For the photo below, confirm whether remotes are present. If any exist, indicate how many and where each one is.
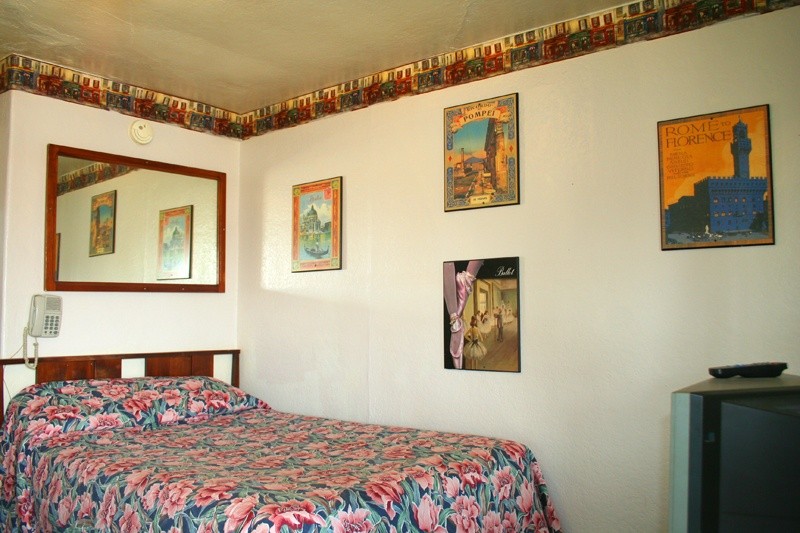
[709,362,788,379]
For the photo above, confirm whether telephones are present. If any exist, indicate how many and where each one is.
[28,294,62,338]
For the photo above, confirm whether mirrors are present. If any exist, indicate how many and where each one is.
[45,143,226,293]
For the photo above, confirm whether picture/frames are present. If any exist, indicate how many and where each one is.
[443,256,521,373]
[442,92,521,212]
[291,176,343,273]
[656,104,776,252]
[89,189,117,257]
[156,204,194,280]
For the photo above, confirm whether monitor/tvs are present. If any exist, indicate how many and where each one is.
[670,371,800,533]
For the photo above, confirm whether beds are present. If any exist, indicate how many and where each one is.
[0,348,567,533]
